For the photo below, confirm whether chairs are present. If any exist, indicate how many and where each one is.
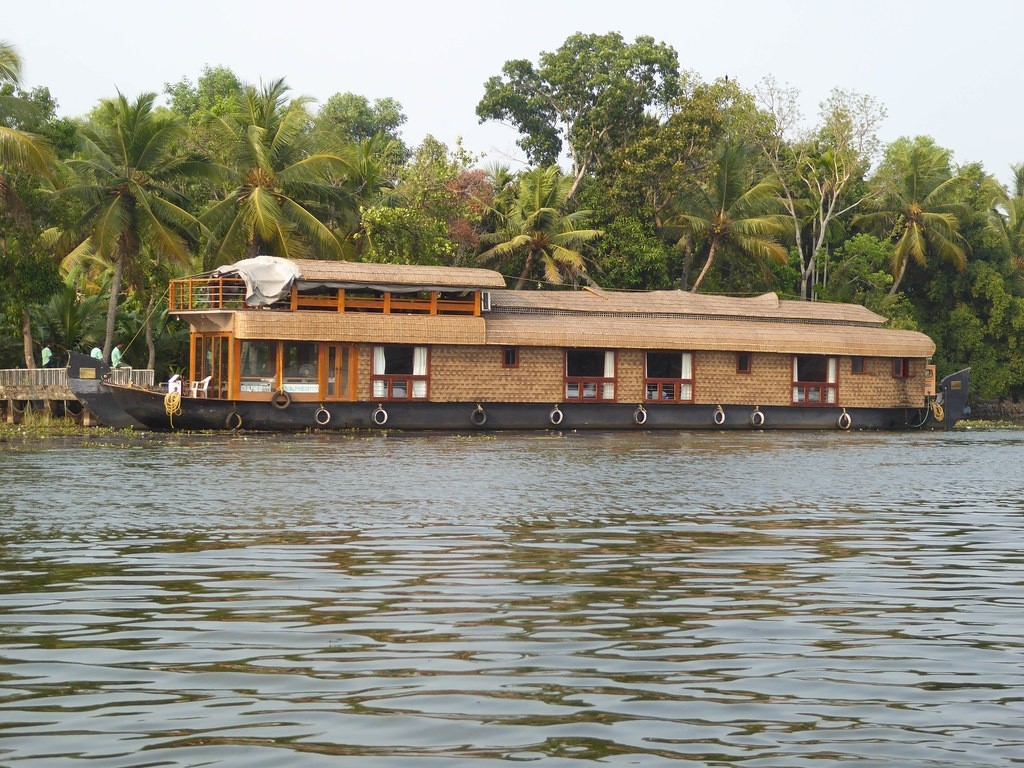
[188,376,213,398]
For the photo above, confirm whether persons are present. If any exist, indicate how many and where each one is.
[42,343,61,369]
[111,342,130,369]
[91,344,104,361]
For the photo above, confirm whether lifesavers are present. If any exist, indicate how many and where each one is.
[224,413,243,430]
[711,410,726,424]
[549,409,564,426]
[471,408,488,427]
[371,410,388,425]
[271,390,292,409]
[836,413,852,430]
[633,407,648,425]
[750,411,765,426]
[313,408,332,426]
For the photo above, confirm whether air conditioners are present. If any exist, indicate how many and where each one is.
[481,292,491,312]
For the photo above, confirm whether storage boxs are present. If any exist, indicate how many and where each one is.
[168,374,183,396]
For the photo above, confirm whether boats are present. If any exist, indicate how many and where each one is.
[53,255,972,431]
